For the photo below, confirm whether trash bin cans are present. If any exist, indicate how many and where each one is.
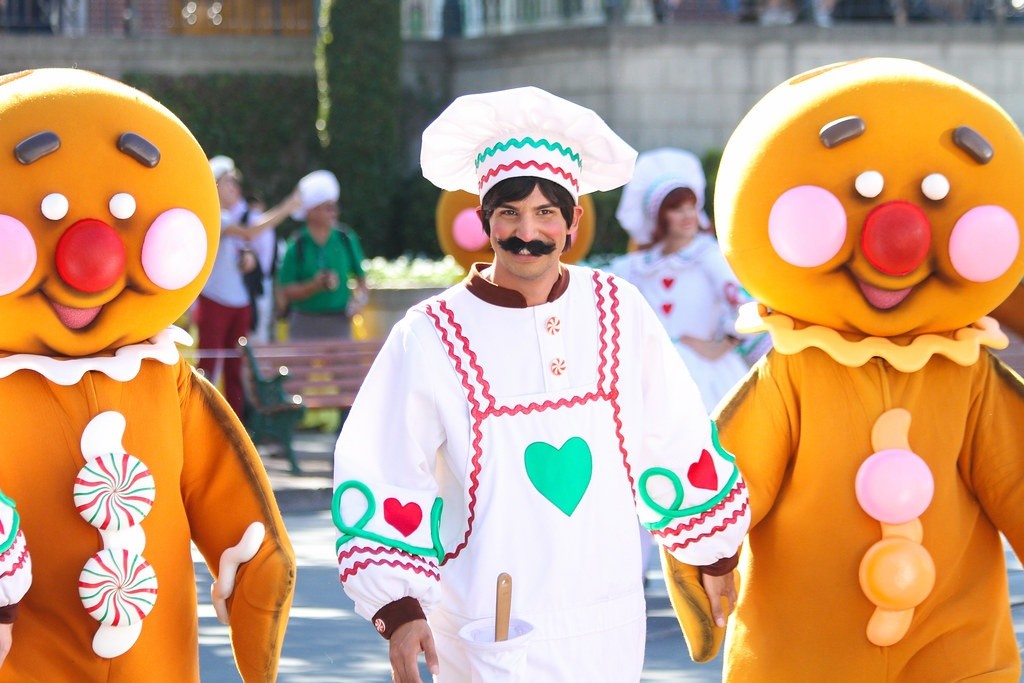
[367,287,449,347]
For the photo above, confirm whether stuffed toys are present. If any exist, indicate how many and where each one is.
[434,186,595,286]
[0,64,298,683]
[660,58,1024,683]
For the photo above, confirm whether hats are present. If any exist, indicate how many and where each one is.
[421,87,640,206]
[615,149,709,245]
[209,156,235,183]
[290,169,340,221]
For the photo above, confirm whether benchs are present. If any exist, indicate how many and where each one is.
[236,335,388,476]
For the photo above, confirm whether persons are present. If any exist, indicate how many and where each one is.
[189,145,369,430]
[604,147,774,422]
[334,87,751,683]
